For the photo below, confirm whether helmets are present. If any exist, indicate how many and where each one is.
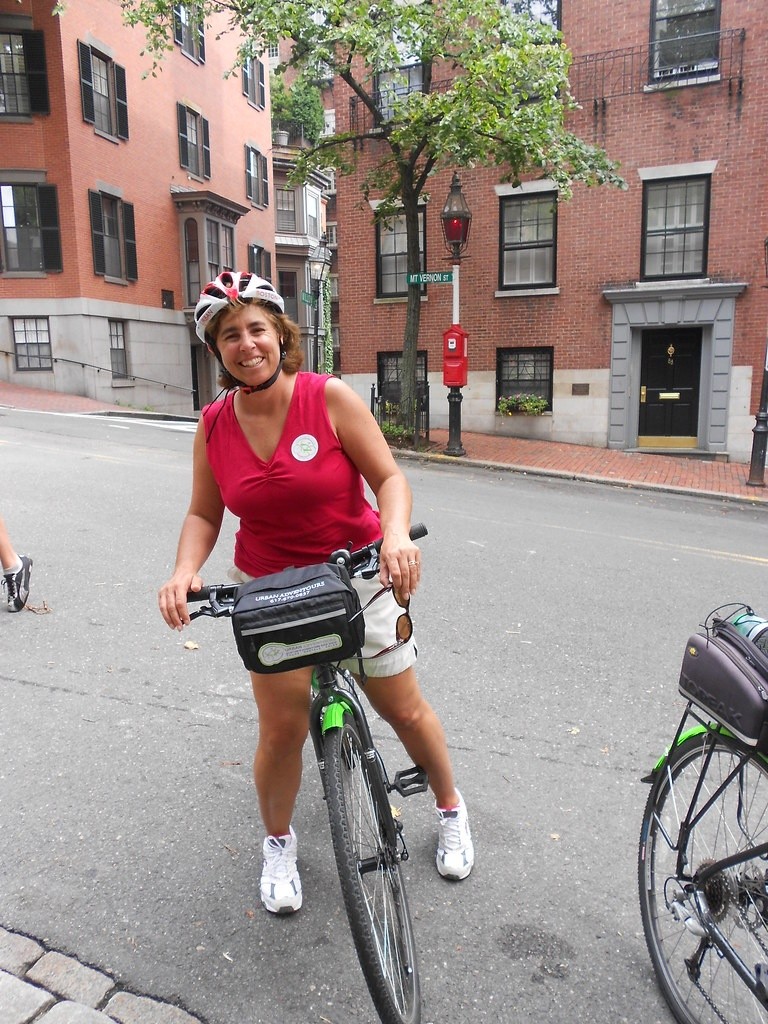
[195,271,287,342]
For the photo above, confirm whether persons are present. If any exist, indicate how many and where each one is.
[159,270,475,915]
[0,516,33,612]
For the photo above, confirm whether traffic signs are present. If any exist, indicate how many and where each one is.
[301,290,316,308]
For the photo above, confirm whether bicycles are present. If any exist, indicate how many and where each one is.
[638,603,768,1024]
[178,521,430,1023]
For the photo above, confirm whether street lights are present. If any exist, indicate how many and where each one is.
[441,169,471,456]
[309,231,334,373]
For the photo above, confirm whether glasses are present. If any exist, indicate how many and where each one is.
[348,583,413,659]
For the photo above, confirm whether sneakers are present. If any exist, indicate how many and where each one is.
[260,824,303,914]
[1,554,33,612]
[434,788,475,881]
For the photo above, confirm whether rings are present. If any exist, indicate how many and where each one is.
[409,561,416,565]
